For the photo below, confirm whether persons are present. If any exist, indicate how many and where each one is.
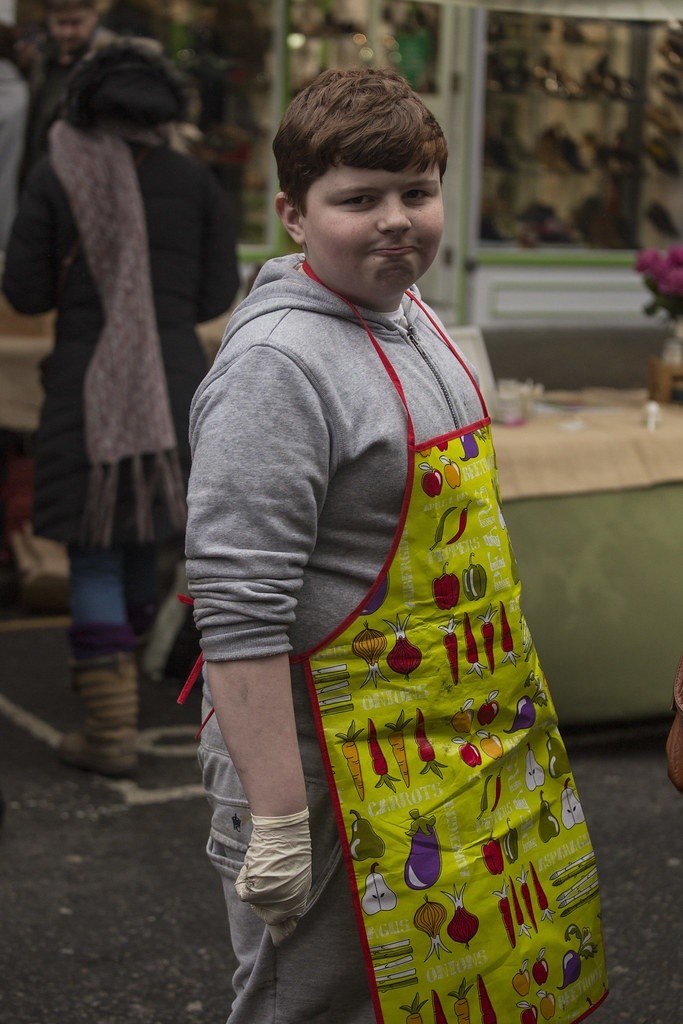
[0,34,238,780]
[179,58,615,1024]
[0,0,253,300]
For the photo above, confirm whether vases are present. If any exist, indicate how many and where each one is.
[661,319,683,365]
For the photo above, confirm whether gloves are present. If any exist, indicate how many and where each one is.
[233,809,312,924]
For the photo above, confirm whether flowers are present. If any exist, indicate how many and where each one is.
[634,247,683,321]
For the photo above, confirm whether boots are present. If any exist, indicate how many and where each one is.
[60,656,140,771]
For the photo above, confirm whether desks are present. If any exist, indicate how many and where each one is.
[489,388,683,725]
